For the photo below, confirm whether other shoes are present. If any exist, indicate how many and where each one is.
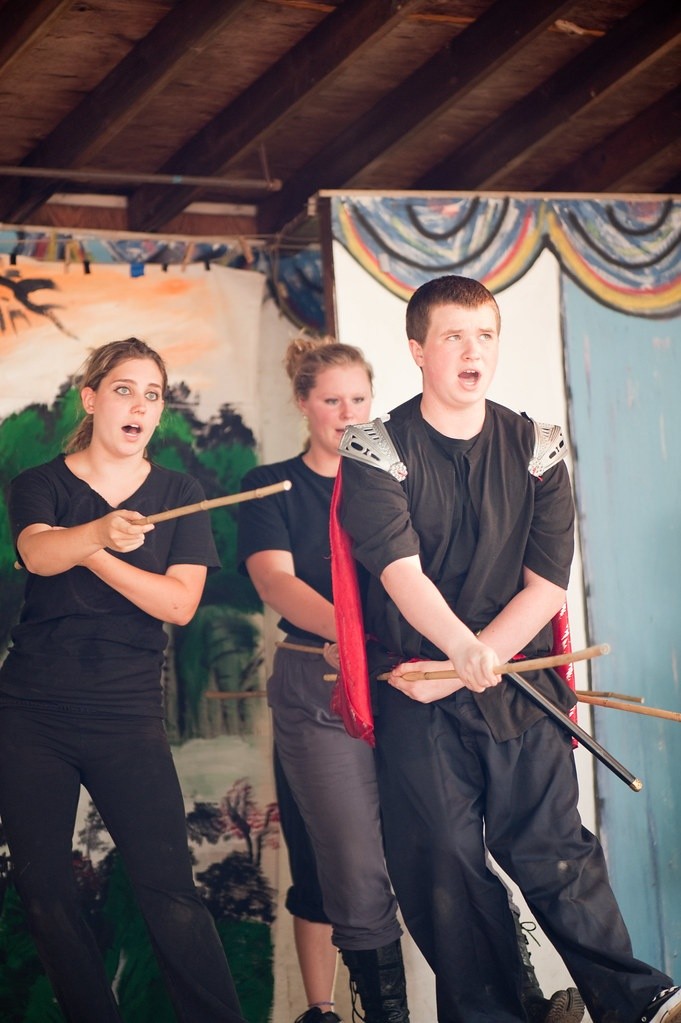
[294,1007,340,1023]
[638,986,681,1023]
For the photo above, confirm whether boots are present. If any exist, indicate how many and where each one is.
[339,945,409,1023]
[510,910,585,1023]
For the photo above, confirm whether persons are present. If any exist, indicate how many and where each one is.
[0,338,245,1023]
[236,336,582,1023]
[333,276,681,1023]
